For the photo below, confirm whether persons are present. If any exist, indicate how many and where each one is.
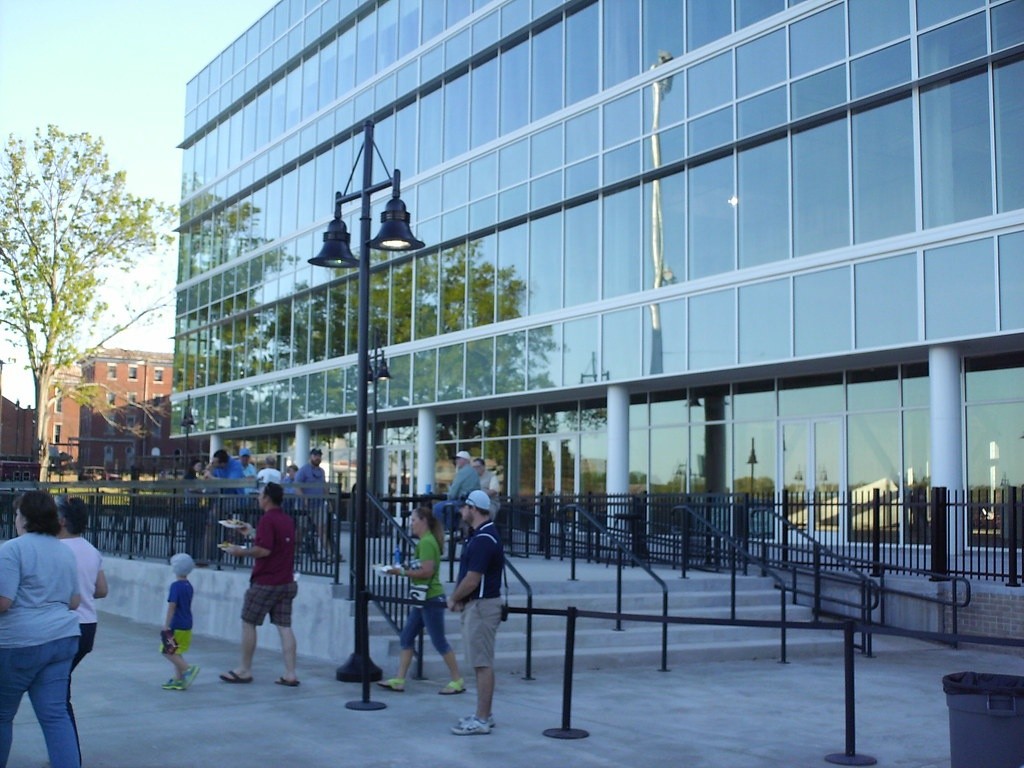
[161,553,201,690]
[183,448,343,567]
[448,490,503,736]
[109,457,121,480]
[219,482,302,686]
[131,457,143,494]
[432,451,500,541]
[150,458,158,492]
[375,507,466,695]
[0,490,109,768]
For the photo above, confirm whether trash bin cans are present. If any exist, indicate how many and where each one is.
[944,671,1024,768]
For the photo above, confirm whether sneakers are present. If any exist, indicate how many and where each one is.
[162,678,185,690]
[450,716,490,735]
[181,664,201,689]
[459,713,495,728]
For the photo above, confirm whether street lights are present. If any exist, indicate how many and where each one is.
[307,122,427,685]
[181,394,195,479]
[366,328,393,538]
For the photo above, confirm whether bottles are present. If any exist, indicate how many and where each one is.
[395,545,401,565]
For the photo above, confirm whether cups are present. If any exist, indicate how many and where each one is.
[426,484,431,495]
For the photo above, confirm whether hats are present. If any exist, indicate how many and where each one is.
[465,489,492,511]
[239,447,250,458]
[310,448,323,456]
[449,450,471,461]
[170,553,194,577]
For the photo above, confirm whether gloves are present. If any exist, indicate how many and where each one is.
[161,629,178,655]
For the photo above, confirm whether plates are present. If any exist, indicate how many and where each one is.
[216,543,247,552]
[218,519,247,529]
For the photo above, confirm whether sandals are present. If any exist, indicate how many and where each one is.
[438,678,467,694]
[375,678,405,691]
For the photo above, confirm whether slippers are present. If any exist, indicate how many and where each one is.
[219,670,253,683]
[274,677,300,687]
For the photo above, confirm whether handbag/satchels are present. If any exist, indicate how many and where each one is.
[501,604,508,621]
